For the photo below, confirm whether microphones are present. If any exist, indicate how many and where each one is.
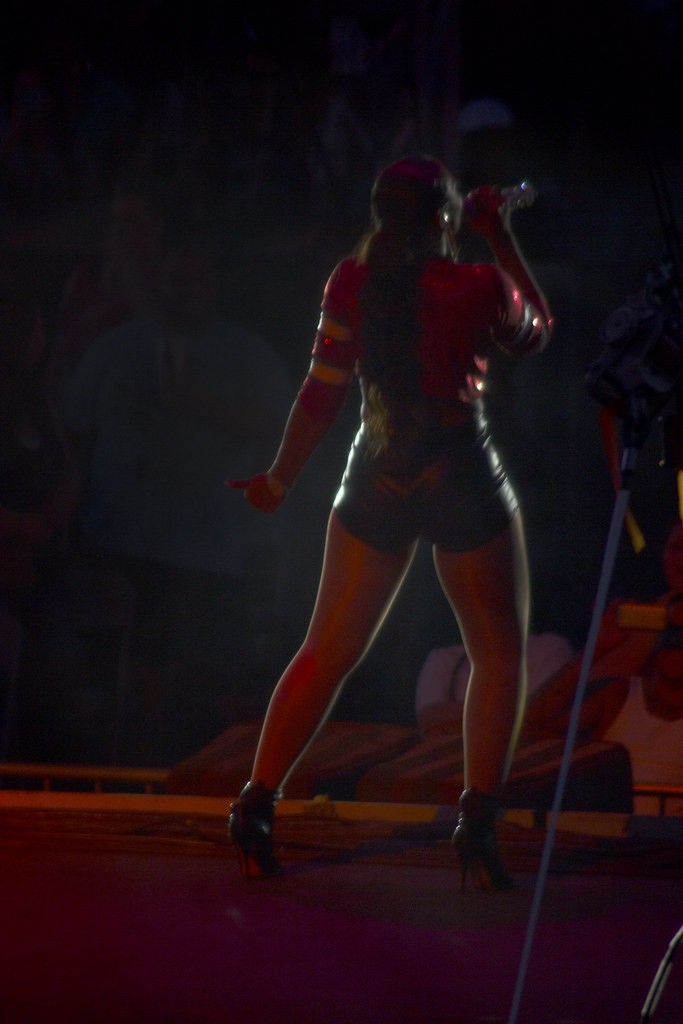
[505,183,536,211]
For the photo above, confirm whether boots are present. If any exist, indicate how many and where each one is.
[448,786,516,898]
[228,779,284,883]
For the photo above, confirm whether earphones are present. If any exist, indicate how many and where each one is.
[443,212,452,223]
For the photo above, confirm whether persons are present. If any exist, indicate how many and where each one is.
[0,0,683,817]
[224,157,550,894]
